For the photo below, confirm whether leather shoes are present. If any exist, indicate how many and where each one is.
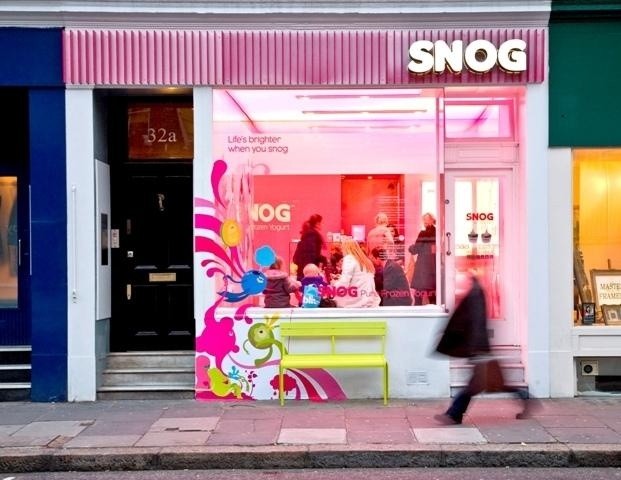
[434,414,458,425]
[516,399,543,419]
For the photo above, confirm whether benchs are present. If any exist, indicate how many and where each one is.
[276,321,390,406]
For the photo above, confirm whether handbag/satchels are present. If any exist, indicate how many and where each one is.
[485,359,518,392]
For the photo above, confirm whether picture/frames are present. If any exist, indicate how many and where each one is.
[581,269,621,326]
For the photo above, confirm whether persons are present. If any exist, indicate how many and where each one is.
[292,211,414,308]
[262,256,302,308]
[431,269,547,426]
[408,212,437,306]
